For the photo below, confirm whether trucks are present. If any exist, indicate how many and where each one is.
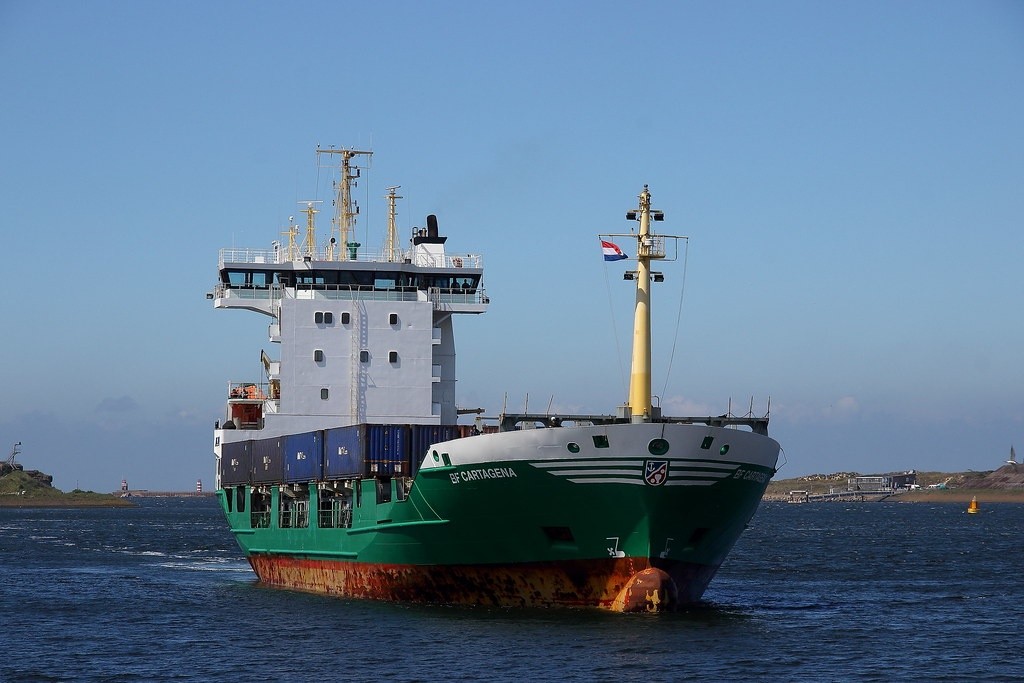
[911,485,938,491]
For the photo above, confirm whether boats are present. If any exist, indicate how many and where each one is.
[214,133,788,614]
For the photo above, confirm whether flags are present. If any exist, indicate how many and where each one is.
[602,240,629,262]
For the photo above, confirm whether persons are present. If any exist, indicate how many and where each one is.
[451,279,470,293]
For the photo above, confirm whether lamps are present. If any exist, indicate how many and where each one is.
[650,274,664,282]
[626,212,640,221]
[206,293,214,300]
[650,213,664,221]
[623,274,636,280]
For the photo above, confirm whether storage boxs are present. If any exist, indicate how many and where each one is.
[220,425,498,486]
